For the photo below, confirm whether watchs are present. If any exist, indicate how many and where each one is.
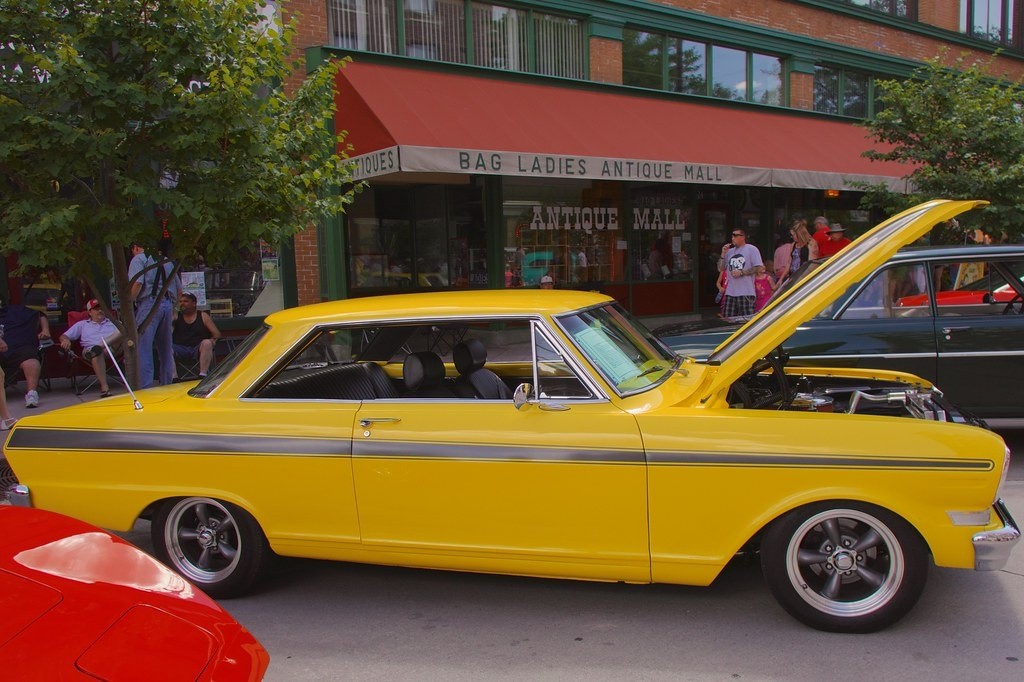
[740,269,744,277]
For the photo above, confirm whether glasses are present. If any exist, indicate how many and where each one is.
[732,233,743,236]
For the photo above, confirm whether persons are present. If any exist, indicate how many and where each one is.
[128,240,182,389]
[0,304,52,433]
[171,291,223,383]
[516,236,677,288]
[540,276,553,289]
[59,298,124,399]
[716,215,992,319]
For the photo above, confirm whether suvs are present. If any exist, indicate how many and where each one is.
[660,243,1024,424]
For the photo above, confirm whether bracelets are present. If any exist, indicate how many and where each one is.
[720,256,726,259]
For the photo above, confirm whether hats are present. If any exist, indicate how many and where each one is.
[825,223,845,235]
[86,298,100,311]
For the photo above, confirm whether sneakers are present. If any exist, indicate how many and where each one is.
[24,390,39,407]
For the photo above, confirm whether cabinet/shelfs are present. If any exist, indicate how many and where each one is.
[517,226,614,284]
[206,298,233,317]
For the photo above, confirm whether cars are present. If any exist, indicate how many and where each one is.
[2,195,1024,634]
[1,505,273,682]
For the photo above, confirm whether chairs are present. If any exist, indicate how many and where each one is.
[403,351,457,397]
[452,338,514,401]
[4,309,216,396]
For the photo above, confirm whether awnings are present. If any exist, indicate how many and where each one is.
[329,58,1003,197]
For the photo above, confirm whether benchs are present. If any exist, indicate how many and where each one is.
[256,361,403,400]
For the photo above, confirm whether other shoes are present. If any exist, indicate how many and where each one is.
[100,390,112,398]
[82,345,102,361]
[0,418,17,430]
[198,374,205,379]
[172,378,181,383]
